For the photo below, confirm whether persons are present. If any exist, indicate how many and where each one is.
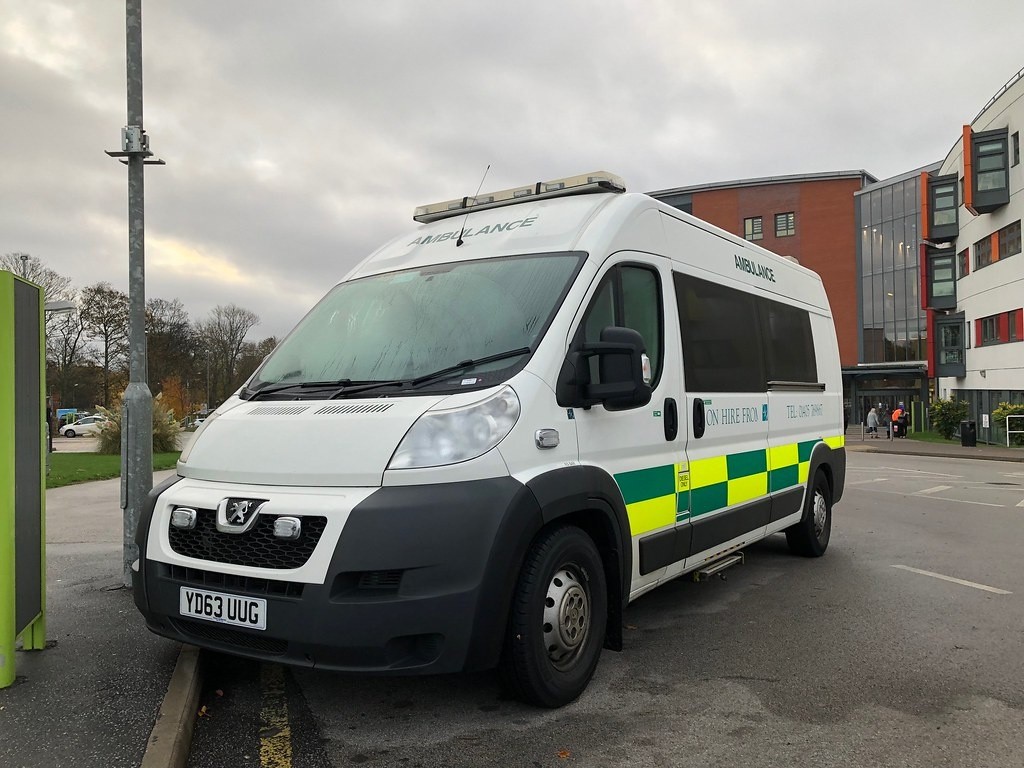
[867,408,880,438]
[884,405,909,440]
[844,408,850,434]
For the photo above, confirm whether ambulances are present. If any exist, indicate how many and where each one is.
[131,163,848,710]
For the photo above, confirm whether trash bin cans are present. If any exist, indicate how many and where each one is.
[961,420,977,447]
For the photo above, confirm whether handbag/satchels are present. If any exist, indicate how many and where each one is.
[866,426,871,433]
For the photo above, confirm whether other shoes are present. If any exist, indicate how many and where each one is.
[875,436,880,438]
[900,436,905,439]
[871,436,873,438]
[887,437,889,439]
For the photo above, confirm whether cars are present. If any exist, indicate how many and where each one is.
[59,415,113,438]
[188,418,205,429]
